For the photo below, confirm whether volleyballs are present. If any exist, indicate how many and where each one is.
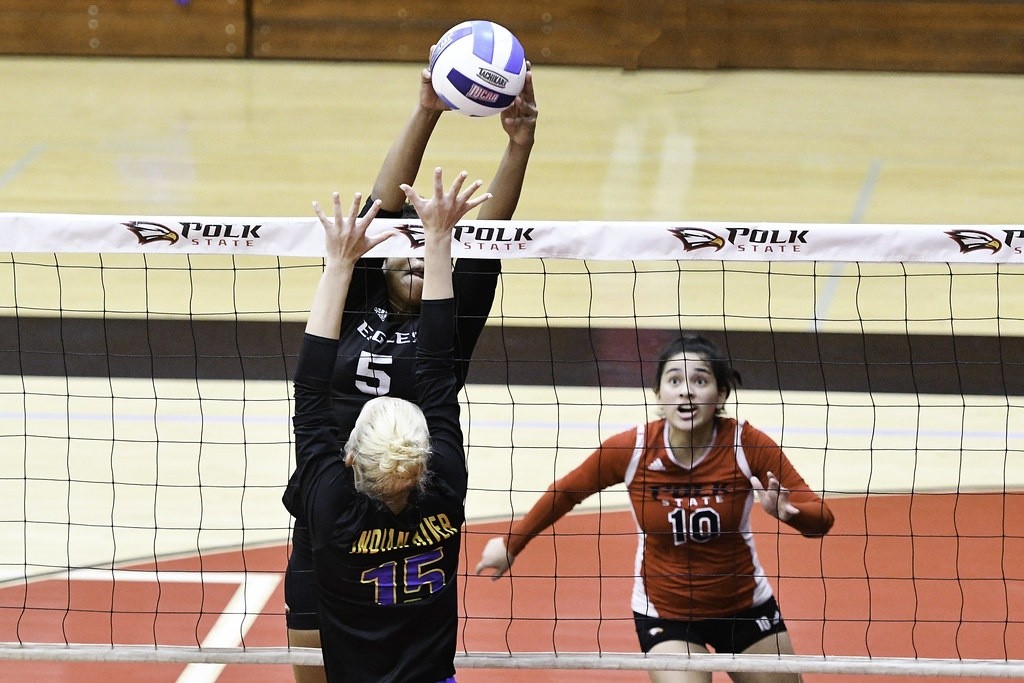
[429,21,527,118]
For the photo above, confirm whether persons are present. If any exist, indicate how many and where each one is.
[283,46,538,683]
[476,335,834,683]
[294,167,493,681]
